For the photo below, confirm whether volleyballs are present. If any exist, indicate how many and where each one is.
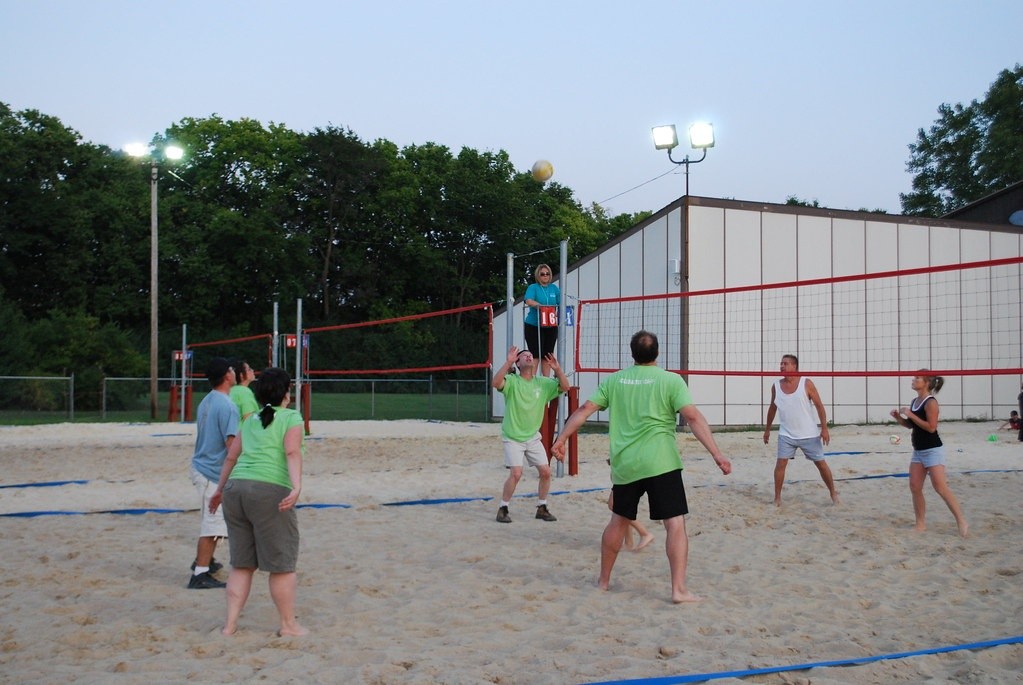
[532,159,553,183]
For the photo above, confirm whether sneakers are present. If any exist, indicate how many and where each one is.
[496,506,512,522]
[191,556,223,573]
[535,505,557,521]
[187,571,227,589]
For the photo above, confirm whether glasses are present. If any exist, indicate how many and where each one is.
[538,271,551,276]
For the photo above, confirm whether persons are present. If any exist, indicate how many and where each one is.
[209,367,309,635]
[524,265,560,377]
[229,361,259,429]
[607,489,655,552]
[1009,410,1020,431]
[551,330,732,603]
[188,358,239,588]
[1018,383,1023,441]
[764,355,839,507]
[890,369,968,538]
[492,346,570,522]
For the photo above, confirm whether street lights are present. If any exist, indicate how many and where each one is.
[652,121,716,432]
[120,140,185,418]
